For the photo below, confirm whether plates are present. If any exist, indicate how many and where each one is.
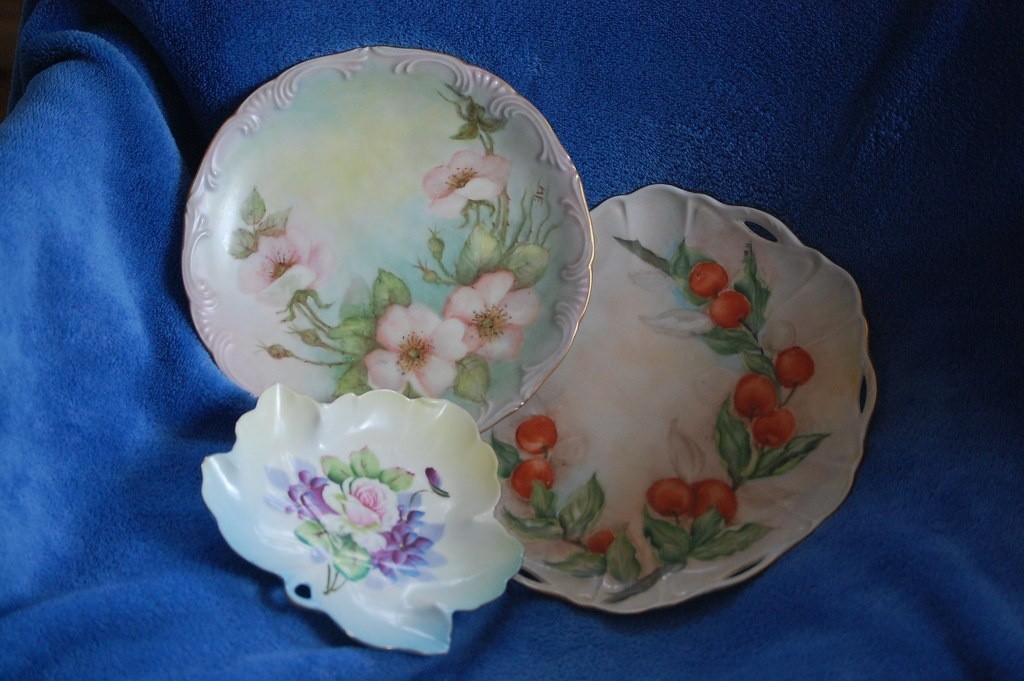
[480,184,877,615]
[199,384,526,653]
[180,45,595,433]
